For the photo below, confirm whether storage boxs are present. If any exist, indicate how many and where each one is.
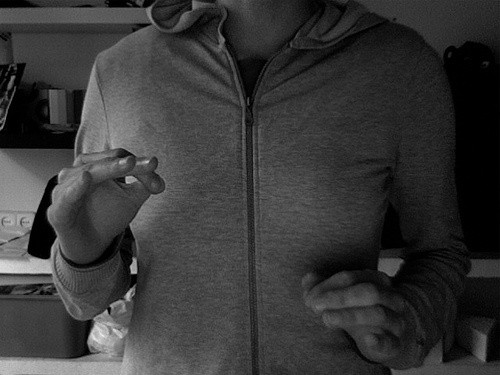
[0,285,89,356]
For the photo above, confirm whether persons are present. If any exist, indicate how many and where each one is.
[46,0,472,375]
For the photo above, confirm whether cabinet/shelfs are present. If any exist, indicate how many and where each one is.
[378,257,500,375]
[0,0,153,150]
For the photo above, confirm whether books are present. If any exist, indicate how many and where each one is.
[40,89,87,125]
